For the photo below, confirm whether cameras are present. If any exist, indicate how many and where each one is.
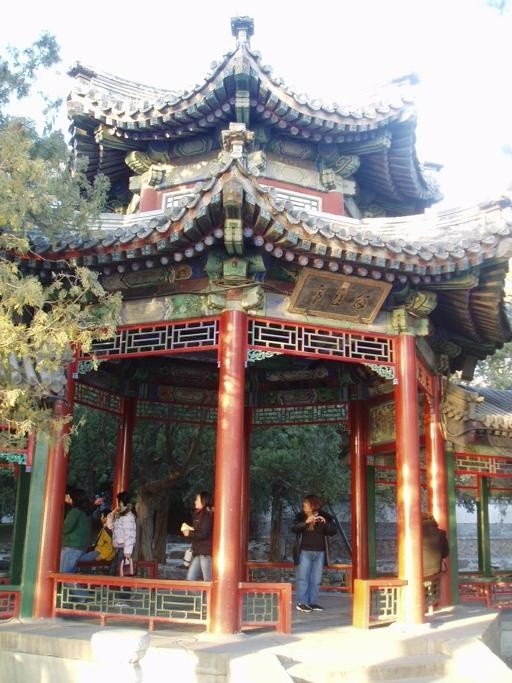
[313,517,324,526]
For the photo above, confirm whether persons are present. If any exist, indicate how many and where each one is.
[58,487,93,610]
[106,492,138,602]
[421,512,450,617]
[180,489,214,618]
[77,506,116,561]
[291,493,337,611]
[80,497,105,514]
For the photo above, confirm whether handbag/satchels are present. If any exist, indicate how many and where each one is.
[118,572,143,597]
[182,548,194,567]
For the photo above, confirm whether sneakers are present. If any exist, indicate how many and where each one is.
[296,603,326,613]
